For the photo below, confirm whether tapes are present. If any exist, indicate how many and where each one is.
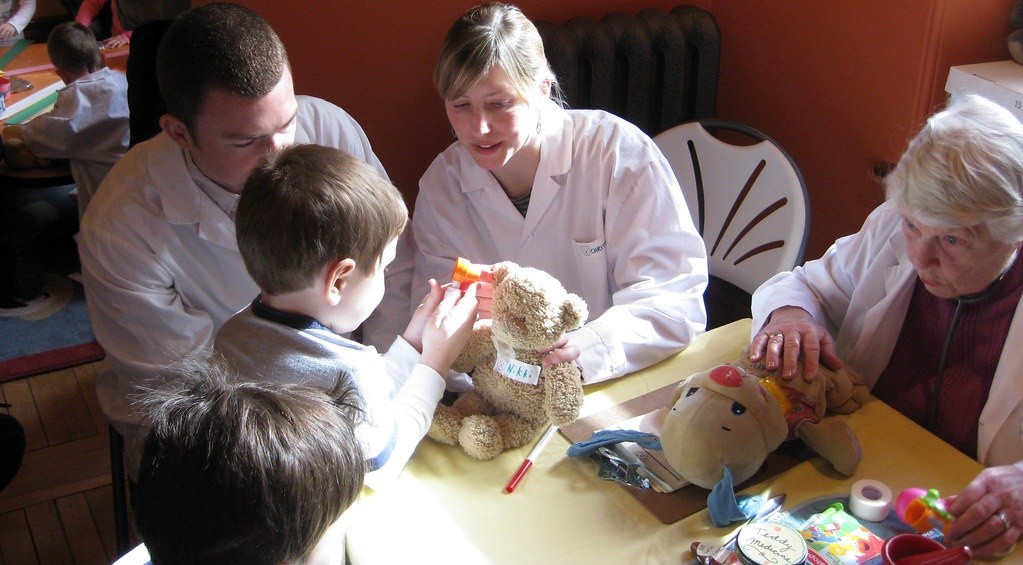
[848,479,892,522]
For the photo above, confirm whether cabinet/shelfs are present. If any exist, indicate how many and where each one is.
[716,0,1023,267]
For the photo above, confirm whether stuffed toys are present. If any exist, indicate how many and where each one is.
[659,350,867,489]
[426,261,589,460]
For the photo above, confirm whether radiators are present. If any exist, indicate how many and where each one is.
[537,4,720,137]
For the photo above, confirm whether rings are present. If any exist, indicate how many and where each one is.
[770,333,783,337]
[995,512,1010,529]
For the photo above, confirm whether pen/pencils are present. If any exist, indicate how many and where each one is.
[506,424,561,492]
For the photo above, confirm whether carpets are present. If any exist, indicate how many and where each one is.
[0,273,107,384]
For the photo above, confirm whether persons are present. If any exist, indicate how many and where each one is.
[78,0,132,48]
[77,7,410,539]
[0,0,37,40]
[130,363,365,565]
[411,7,709,385]
[750,94,1023,557]
[213,144,480,490]
[0,22,130,307]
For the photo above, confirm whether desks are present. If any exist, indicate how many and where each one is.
[349,318,1023,565]
[0,163,75,188]
[0,42,129,77]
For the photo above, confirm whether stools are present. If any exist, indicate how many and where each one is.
[96,360,152,555]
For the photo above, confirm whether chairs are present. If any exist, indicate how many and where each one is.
[651,120,810,331]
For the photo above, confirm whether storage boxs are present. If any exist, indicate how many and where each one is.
[944,60,1023,123]
